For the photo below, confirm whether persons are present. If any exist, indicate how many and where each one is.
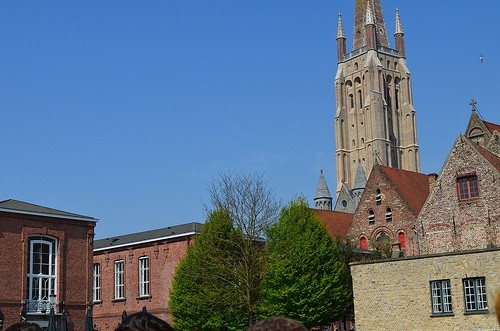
[247,317,308,331]
[4,322,44,331]
[114,311,174,331]
